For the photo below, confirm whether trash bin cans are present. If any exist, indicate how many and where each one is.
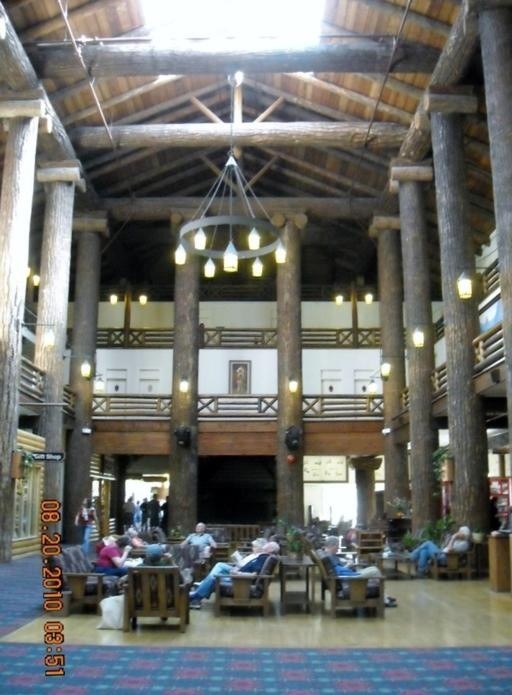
[486,530,512,592]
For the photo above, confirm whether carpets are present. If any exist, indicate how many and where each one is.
[0,556,511,694]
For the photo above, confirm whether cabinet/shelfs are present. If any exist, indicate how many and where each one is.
[357,530,384,567]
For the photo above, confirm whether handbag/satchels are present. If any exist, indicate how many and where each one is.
[97,593,125,630]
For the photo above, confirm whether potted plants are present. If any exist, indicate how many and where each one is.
[473,525,484,541]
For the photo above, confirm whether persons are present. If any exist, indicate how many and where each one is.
[408,525,472,579]
[302,516,398,607]
[75,493,281,610]
[489,495,502,530]
[236,367,245,393]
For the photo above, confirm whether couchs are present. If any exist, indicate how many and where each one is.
[42,544,122,615]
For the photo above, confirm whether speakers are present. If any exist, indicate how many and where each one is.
[284,425,304,450]
[173,426,190,447]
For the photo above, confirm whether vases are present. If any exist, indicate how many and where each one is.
[47,591,73,616]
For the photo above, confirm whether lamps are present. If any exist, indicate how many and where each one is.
[457,266,500,299]
[174,72,289,277]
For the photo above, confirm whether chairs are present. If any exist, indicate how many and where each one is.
[124,565,193,631]
[310,548,386,615]
[213,556,278,617]
[428,548,475,578]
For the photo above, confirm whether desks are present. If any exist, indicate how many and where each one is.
[280,558,318,614]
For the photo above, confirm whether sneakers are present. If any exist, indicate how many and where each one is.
[384,596,397,607]
[188,591,202,609]
